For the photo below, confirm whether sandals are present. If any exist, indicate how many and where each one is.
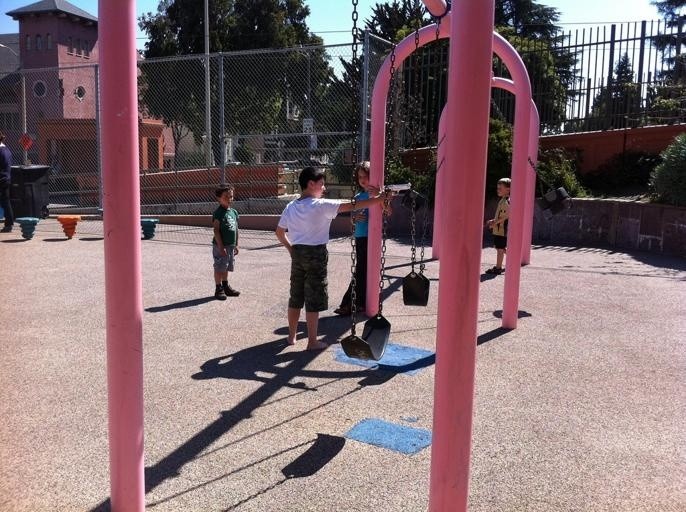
[485,266,506,276]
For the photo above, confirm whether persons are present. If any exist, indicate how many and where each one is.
[333,160,383,317]
[486,176,511,274]
[212,182,242,301]
[275,165,392,352]
[0,133,14,233]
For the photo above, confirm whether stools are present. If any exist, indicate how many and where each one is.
[141,218,160,239]
[16,216,39,241]
[57,214,81,239]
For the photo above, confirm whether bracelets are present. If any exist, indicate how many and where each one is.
[374,191,382,198]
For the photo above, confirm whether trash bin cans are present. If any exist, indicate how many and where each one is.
[0,165,50,221]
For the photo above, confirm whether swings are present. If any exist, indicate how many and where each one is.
[402,13,441,305]
[340,0,397,361]
[489,97,573,221]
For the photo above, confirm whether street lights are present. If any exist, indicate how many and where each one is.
[0,43,27,165]
[293,44,310,118]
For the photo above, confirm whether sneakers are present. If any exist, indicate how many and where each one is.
[215,284,240,300]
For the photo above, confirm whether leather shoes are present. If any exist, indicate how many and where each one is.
[0,225,11,233]
[334,302,366,316]
